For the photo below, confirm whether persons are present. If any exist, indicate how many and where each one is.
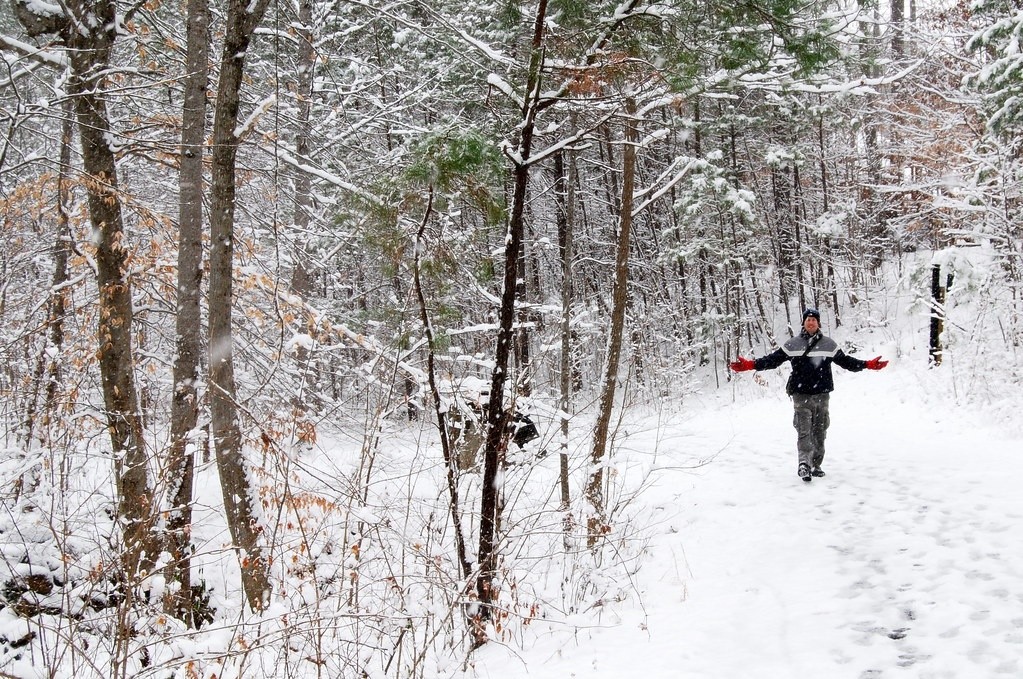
[730,310,889,482]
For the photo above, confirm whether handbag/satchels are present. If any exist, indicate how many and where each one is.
[786,376,800,395]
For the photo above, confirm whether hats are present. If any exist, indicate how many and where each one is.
[801,309,821,328]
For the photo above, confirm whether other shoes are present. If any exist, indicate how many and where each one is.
[798,463,812,481]
[812,469,825,477]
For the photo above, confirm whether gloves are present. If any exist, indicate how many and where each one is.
[866,356,889,370]
[730,356,755,371]
